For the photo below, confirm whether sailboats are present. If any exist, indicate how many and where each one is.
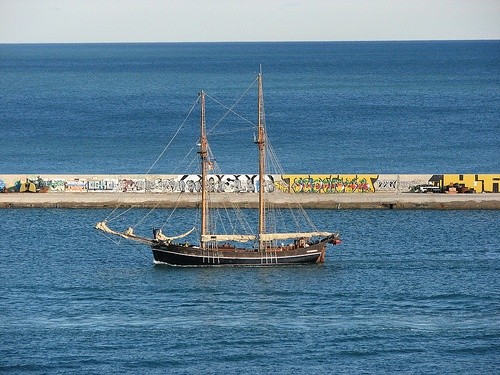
[93,62,340,268]
